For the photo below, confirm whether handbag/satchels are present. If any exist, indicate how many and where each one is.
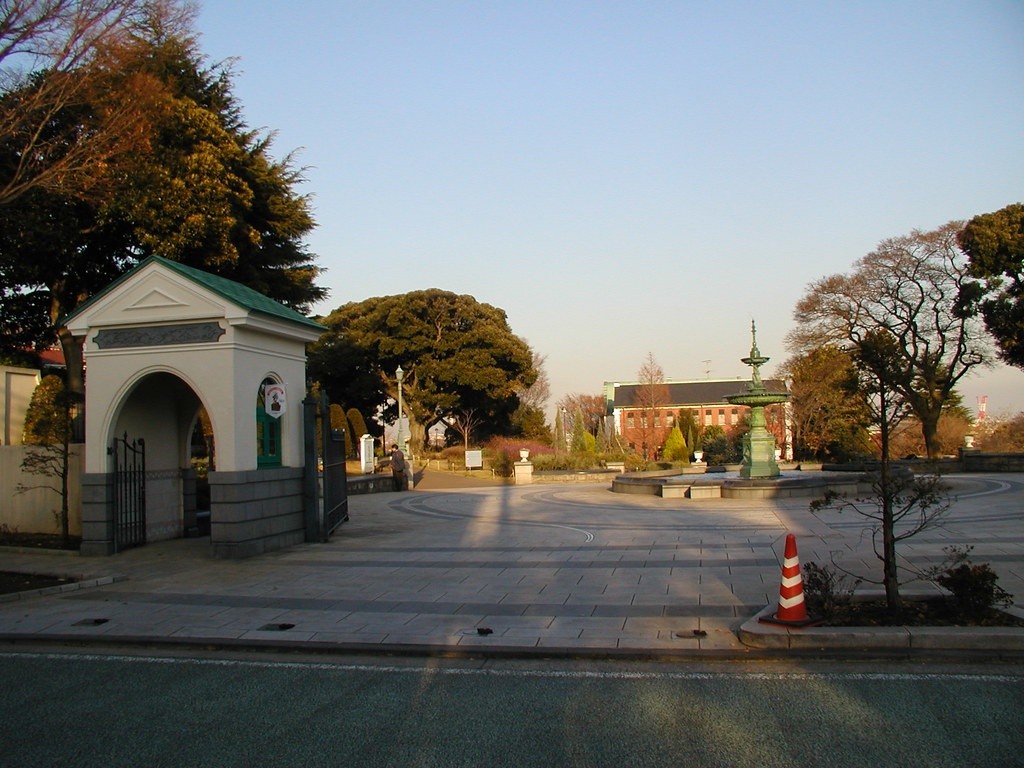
[394,458,405,471]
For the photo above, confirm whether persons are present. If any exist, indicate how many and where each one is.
[391,444,405,491]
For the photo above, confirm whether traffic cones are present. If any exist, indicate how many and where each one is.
[757,532,828,631]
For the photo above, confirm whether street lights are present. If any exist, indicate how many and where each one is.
[395,363,407,451]
[782,374,794,463]
